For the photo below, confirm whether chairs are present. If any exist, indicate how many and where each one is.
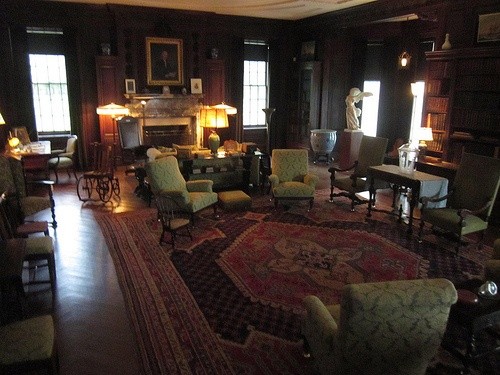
[0,116,500,375]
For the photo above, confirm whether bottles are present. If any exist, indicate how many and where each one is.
[442,34,451,50]
[397,140,420,175]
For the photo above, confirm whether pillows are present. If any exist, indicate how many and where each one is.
[173,143,196,151]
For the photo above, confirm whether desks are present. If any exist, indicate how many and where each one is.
[366,166,449,235]
[441,279,500,375]
[20,141,52,178]
[0,239,26,320]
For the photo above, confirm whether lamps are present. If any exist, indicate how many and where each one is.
[97,102,130,170]
[398,15,411,70]
[199,101,238,157]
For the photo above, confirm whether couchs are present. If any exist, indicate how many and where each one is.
[146,142,259,185]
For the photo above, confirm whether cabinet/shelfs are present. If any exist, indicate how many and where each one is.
[286,60,321,148]
[415,46,500,179]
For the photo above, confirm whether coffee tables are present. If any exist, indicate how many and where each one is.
[181,151,264,187]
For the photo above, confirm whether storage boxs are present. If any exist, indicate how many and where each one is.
[242,143,258,153]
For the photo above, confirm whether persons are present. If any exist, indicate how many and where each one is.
[344,88,373,130]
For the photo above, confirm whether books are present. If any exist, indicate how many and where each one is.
[426,56,500,163]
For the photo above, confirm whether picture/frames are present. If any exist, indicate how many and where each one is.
[299,39,318,61]
[125,78,136,94]
[473,6,500,46]
[13,127,31,145]
[146,37,183,85]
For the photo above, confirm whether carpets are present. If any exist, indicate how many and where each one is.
[95,188,500,375]
